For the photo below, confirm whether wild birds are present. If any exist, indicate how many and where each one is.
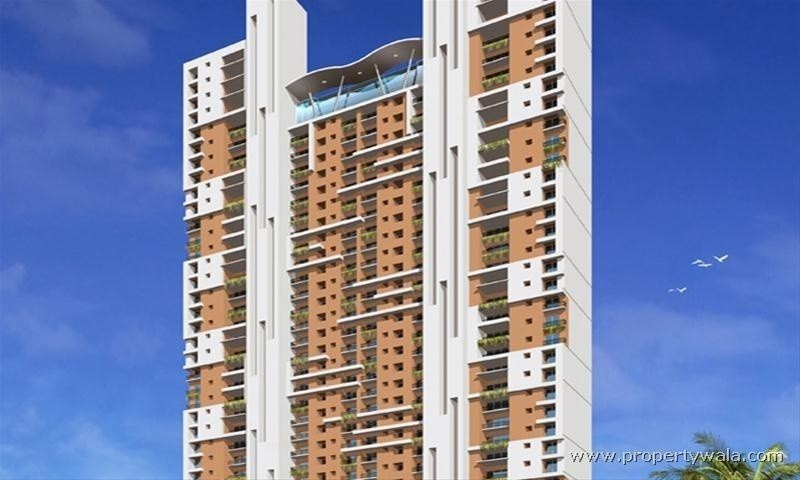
[691,259,704,264]
[676,286,687,294]
[713,254,728,263]
[698,263,712,268]
